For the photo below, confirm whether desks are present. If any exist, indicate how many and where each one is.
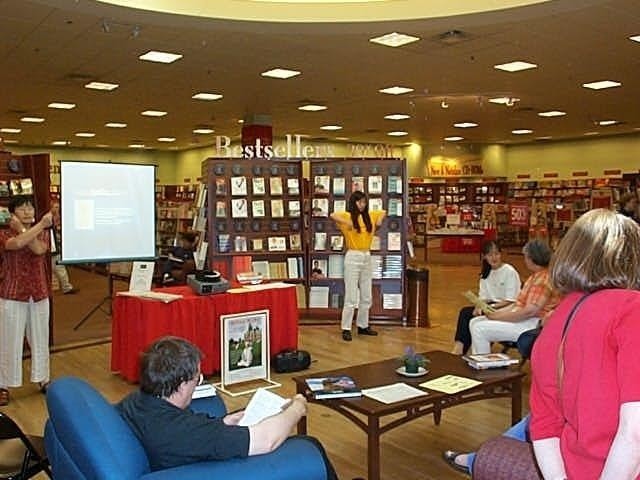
[291,348,526,480]
[112,278,297,385]
[424,226,484,262]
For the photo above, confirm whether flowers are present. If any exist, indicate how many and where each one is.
[397,345,430,367]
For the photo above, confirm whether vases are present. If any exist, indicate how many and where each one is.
[405,361,419,373]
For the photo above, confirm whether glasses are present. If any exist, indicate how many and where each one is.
[16,206,34,213]
[183,376,203,384]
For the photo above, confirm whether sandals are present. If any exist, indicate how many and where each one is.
[442,448,473,472]
[39,379,52,394]
[1,388,10,405]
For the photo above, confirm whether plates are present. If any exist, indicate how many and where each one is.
[397,367,428,376]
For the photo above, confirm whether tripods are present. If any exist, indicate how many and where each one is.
[73,263,114,331]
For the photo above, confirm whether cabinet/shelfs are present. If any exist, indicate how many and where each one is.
[408,182,439,246]
[308,158,410,326]
[505,178,625,204]
[0,153,37,227]
[155,184,208,260]
[201,158,307,311]
[440,184,503,204]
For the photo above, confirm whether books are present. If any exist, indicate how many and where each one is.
[333,199,346,214]
[288,201,301,218]
[536,179,563,232]
[231,199,248,219]
[270,199,284,218]
[506,181,537,200]
[369,236,381,250]
[157,183,208,229]
[269,176,283,196]
[384,254,402,280]
[251,240,262,250]
[366,255,384,280]
[294,285,308,310]
[0,177,34,196]
[252,177,265,196]
[368,175,382,194]
[308,285,344,310]
[381,281,403,310]
[252,200,265,217]
[314,175,330,194]
[156,229,174,256]
[209,256,306,282]
[438,186,468,207]
[562,180,591,197]
[303,375,362,399]
[387,175,402,194]
[0,207,9,224]
[287,178,299,195]
[218,233,231,252]
[350,177,364,195]
[311,198,328,218]
[268,237,286,252]
[215,175,229,196]
[233,236,247,251]
[473,186,508,243]
[313,232,328,251]
[192,228,211,271]
[215,200,227,218]
[409,185,433,246]
[461,353,520,371]
[388,232,401,251]
[332,177,345,198]
[330,235,345,251]
[329,255,344,278]
[369,198,383,213]
[231,176,248,196]
[387,198,403,216]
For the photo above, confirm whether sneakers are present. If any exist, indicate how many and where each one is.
[451,342,464,356]
[343,329,354,342]
[357,326,377,336]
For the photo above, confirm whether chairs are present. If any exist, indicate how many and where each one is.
[0,412,52,480]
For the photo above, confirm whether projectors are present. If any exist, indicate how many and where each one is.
[187,269,229,295]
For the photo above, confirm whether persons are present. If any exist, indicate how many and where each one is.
[313,200,324,217]
[469,240,554,353]
[0,199,53,406]
[312,261,325,279]
[271,239,276,247]
[440,405,532,477]
[322,377,355,392]
[162,231,200,272]
[167,260,195,284]
[471,208,640,480]
[333,237,342,250]
[329,193,386,341]
[315,177,327,192]
[618,192,637,220]
[446,204,450,215]
[225,317,264,372]
[450,205,459,213]
[292,236,299,250]
[48,197,80,294]
[450,239,522,353]
[112,335,366,480]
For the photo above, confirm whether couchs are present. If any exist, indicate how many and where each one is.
[44,377,334,480]
[516,327,542,361]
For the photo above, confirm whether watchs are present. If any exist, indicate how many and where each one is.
[15,227,28,237]
